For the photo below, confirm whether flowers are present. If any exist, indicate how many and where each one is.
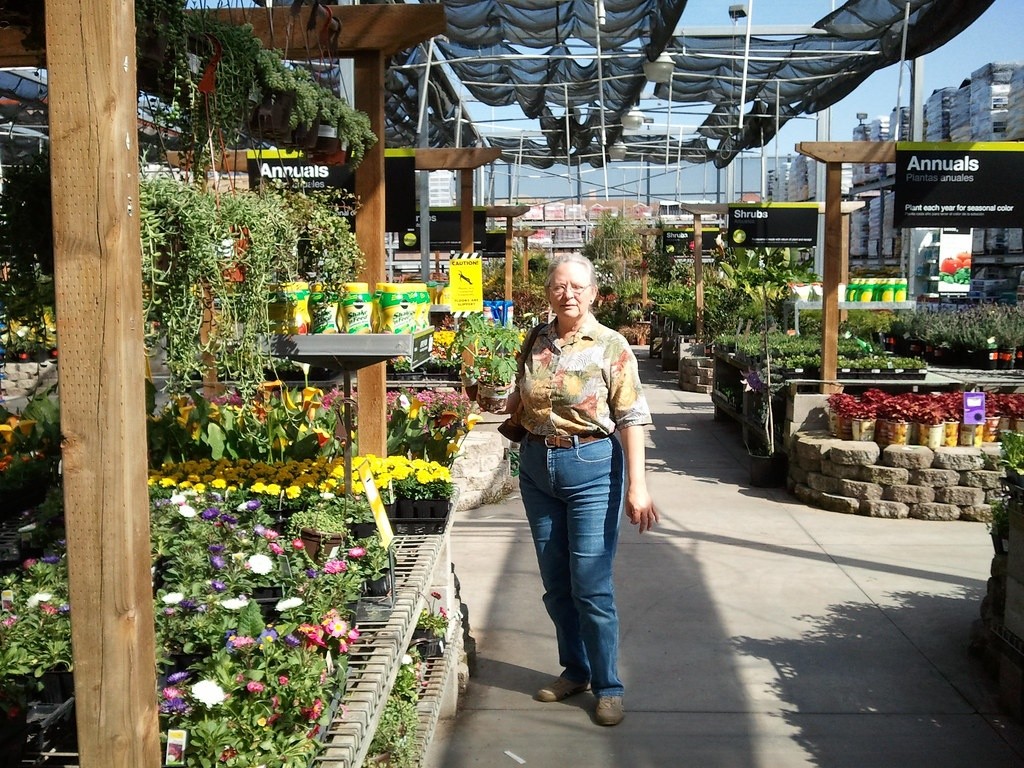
[0,362,476,768]
[431,327,526,354]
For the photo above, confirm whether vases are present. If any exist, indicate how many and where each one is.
[432,499,449,519]
[165,652,205,685]
[415,499,432,518]
[42,670,74,703]
[398,497,415,518]
[383,502,397,519]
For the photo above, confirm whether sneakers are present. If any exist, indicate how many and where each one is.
[596,696,624,725]
[534,677,591,702]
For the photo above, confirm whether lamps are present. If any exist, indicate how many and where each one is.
[641,51,676,83]
[621,105,645,129]
[609,139,627,161]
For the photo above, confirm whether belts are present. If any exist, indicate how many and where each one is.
[525,432,607,448]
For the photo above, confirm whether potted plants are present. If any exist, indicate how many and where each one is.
[0,0,378,362]
[446,311,526,413]
[345,492,379,541]
[364,546,398,596]
[286,509,355,568]
[825,387,1024,449]
[596,244,1024,379]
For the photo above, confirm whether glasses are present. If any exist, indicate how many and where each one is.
[548,284,594,293]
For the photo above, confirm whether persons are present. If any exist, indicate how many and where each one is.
[505,251,660,726]
